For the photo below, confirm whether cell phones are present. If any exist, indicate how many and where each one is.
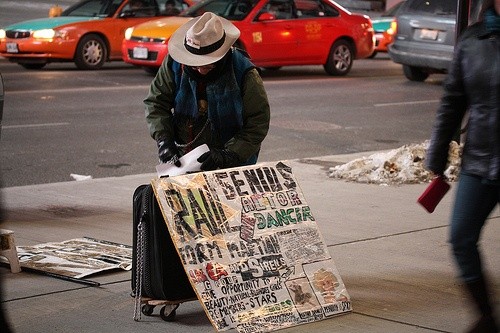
[418,177,450,213]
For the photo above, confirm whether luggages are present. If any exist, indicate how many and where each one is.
[129,182,198,322]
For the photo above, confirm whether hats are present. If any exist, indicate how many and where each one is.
[167,12,240,66]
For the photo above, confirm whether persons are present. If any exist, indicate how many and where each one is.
[417,1,500,333]
[161,0,180,16]
[142,11,270,172]
[130,1,147,16]
[266,3,280,19]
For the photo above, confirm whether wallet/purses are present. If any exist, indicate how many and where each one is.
[417,174,451,213]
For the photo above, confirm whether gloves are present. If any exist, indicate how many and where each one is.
[156,135,183,167]
[197,148,230,171]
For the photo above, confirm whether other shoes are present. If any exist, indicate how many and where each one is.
[465,316,499,333]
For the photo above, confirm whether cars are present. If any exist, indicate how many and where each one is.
[0,0,195,70]
[122,0,375,76]
[367,1,405,59]
[250,0,251,0]
[388,0,500,81]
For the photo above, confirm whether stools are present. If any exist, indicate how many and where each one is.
[0,228,21,273]
